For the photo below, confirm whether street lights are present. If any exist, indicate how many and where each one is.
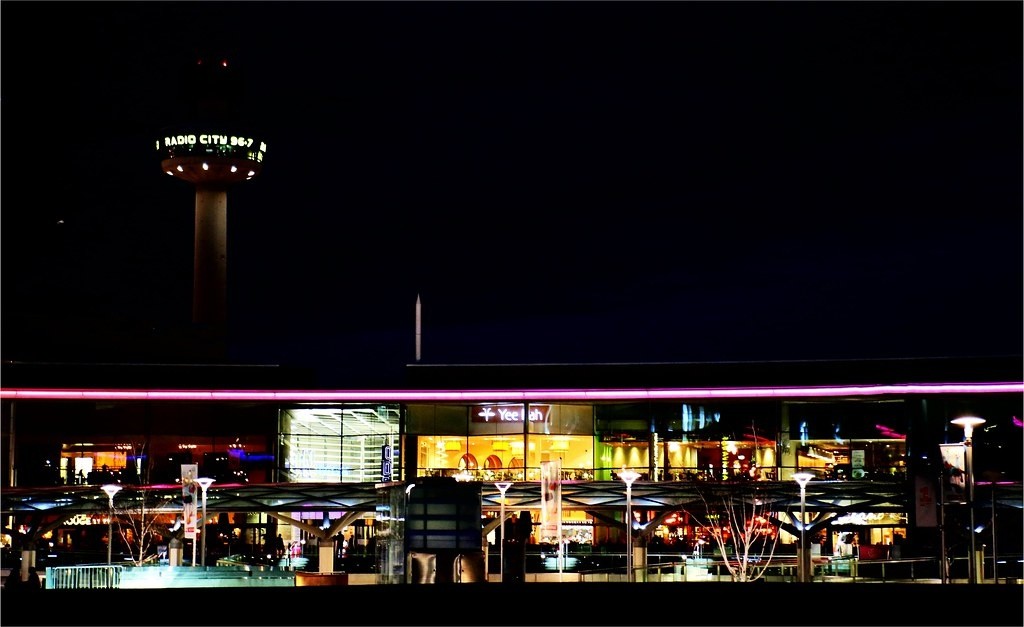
[99,485,123,565]
[617,473,642,581]
[790,473,815,581]
[495,482,513,580]
[952,416,985,585]
[194,478,214,564]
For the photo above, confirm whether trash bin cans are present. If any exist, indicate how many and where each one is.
[169,538,184,567]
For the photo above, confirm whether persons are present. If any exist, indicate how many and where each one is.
[610,466,775,482]
[77,464,126,486]
[261,531,301,556]
[648,528,782,553]
[822,467,905,482]
[334,531,354,555]
